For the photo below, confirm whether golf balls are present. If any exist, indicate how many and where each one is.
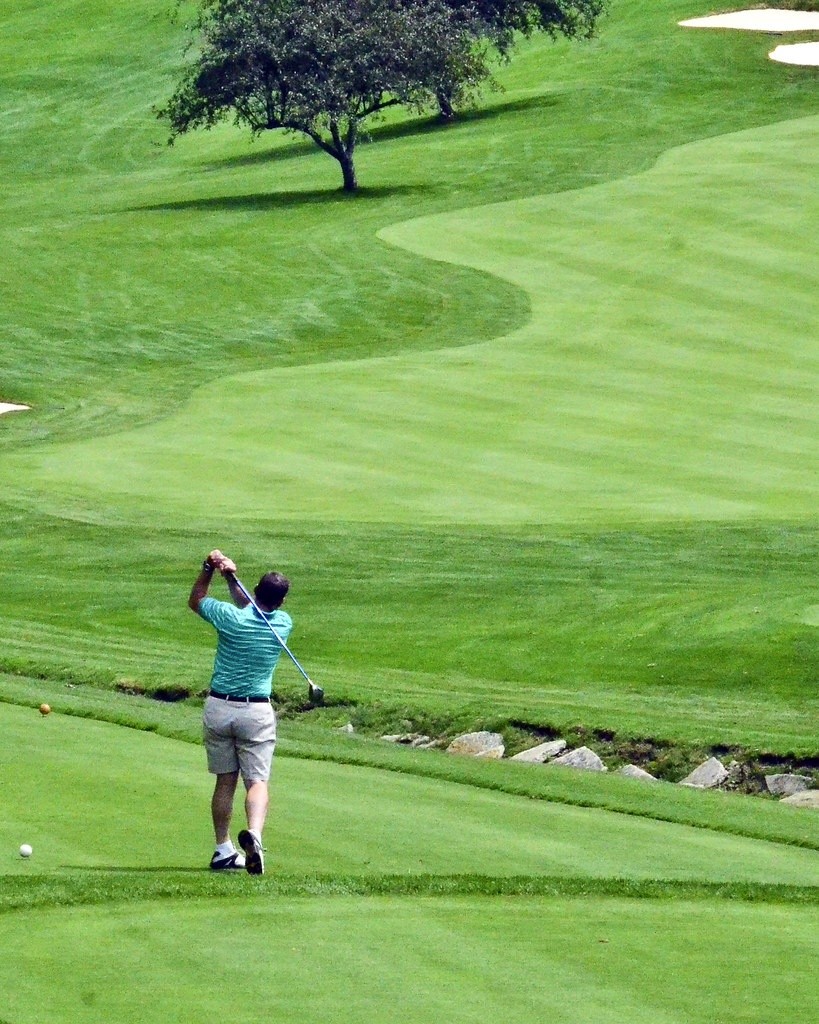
[18,843,34,858]
[38,703,52,715]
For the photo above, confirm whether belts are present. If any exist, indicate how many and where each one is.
[209,690,271,702]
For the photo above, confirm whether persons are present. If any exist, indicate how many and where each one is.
[188,549,291,877]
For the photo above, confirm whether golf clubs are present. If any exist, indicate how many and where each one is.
[230,570,325,706]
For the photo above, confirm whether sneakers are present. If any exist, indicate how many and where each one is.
[209,851,246,869]
[237,829,267,874]
